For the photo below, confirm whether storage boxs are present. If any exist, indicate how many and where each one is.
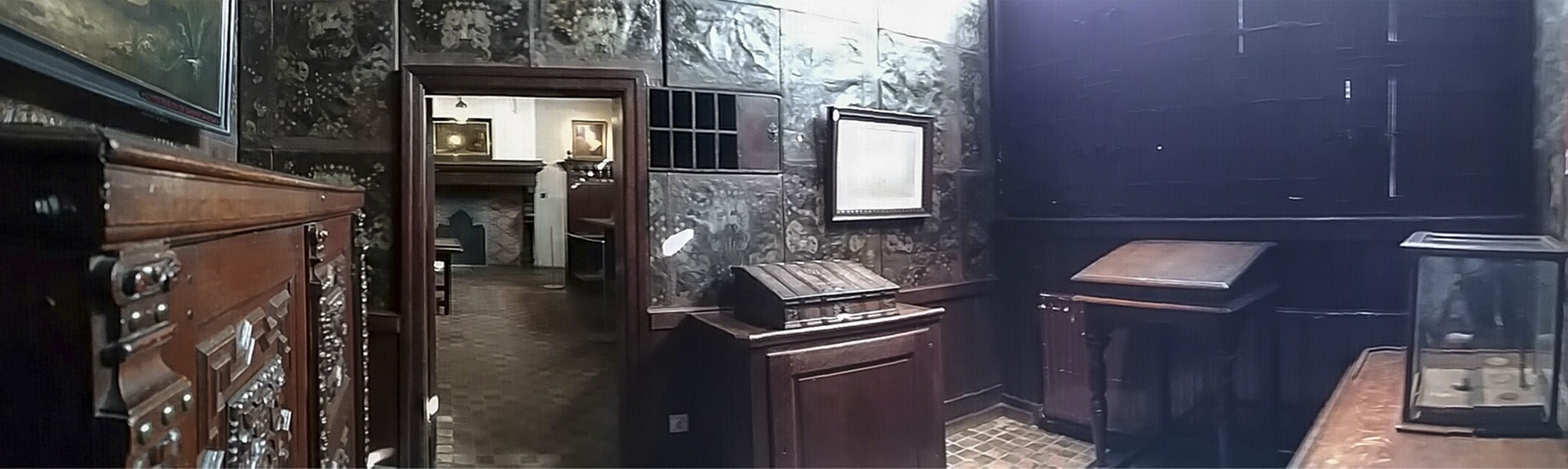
[1395,231,1568,438]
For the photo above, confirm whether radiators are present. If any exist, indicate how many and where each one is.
[1035,288,1274,445]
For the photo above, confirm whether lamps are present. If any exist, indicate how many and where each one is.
[455,97,466,108]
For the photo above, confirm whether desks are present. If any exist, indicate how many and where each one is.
[435,236,464,315]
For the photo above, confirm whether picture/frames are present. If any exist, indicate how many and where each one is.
[430,116,493,160]
[0,0,236,135]
[827,105,933,223]
[570,119,608,160]
